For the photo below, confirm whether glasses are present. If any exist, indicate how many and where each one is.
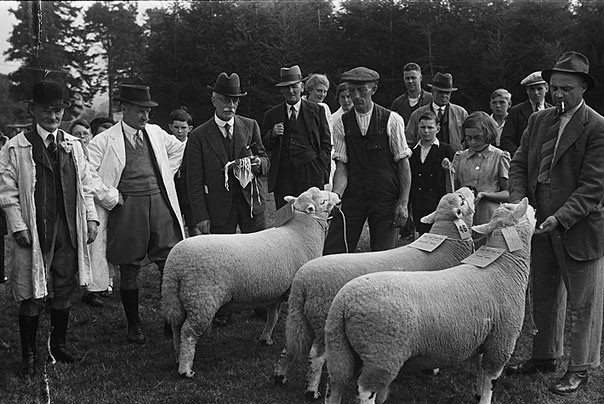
[464,134,484,140]
[73,133,91,138]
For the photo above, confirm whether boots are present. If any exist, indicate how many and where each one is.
[120,287,146,343]
[18,314,38,377]
[49,309,74,364]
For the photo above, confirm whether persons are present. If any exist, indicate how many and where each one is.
[167,105,194,249]
[443,110,513,256]
[487,87,511,145]
[509,52,601,395]
[261,62,332,266]
[389,65,434,127]
[182,73,272,344]
[330,65,412,254]
[88,114,113,131]
[498,69,552,159]
[87,80,187,365]
[0,79,97,381]
[0,132,14,283]
[405,73,471,161]
[406,113,454,242]
[330,84,358,195]
[68,116,103,314]
[300,73,336,196]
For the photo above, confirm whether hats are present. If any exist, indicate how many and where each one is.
[114,84,159,108]
[275,65,308,86]
[427,72,458,91]
[24,81,71,109]
[341,67,380,81]
[541,50,596,91]
[207,71,248,97]
[521,71,546,86]
[90,118,115,134]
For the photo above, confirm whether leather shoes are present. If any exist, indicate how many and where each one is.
[214,313,228,326]
[552,371,587,395]
[507,360,559,374]
[83,293,104,307]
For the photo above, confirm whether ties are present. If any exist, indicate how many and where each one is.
[290,106,297,121]
[47,134,58,165]
[535,103,540,110]
[538,111,561,185]
[135,130,144,151]
[436,107,443,118]
[224,123,231,142]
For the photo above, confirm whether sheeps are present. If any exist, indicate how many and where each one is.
[273,185,476,402]
[324,196,536,404]
[160,186,342,380]
